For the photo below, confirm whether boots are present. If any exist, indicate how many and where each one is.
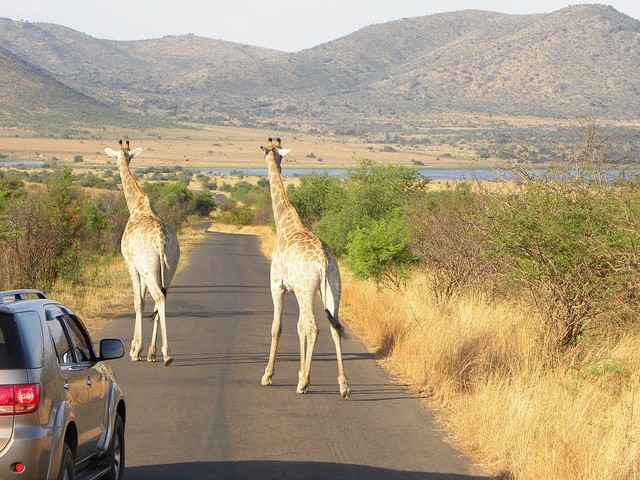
[0,289,131,480]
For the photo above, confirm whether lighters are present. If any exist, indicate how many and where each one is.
[259,136,354,401]
[102,139,182,367]
